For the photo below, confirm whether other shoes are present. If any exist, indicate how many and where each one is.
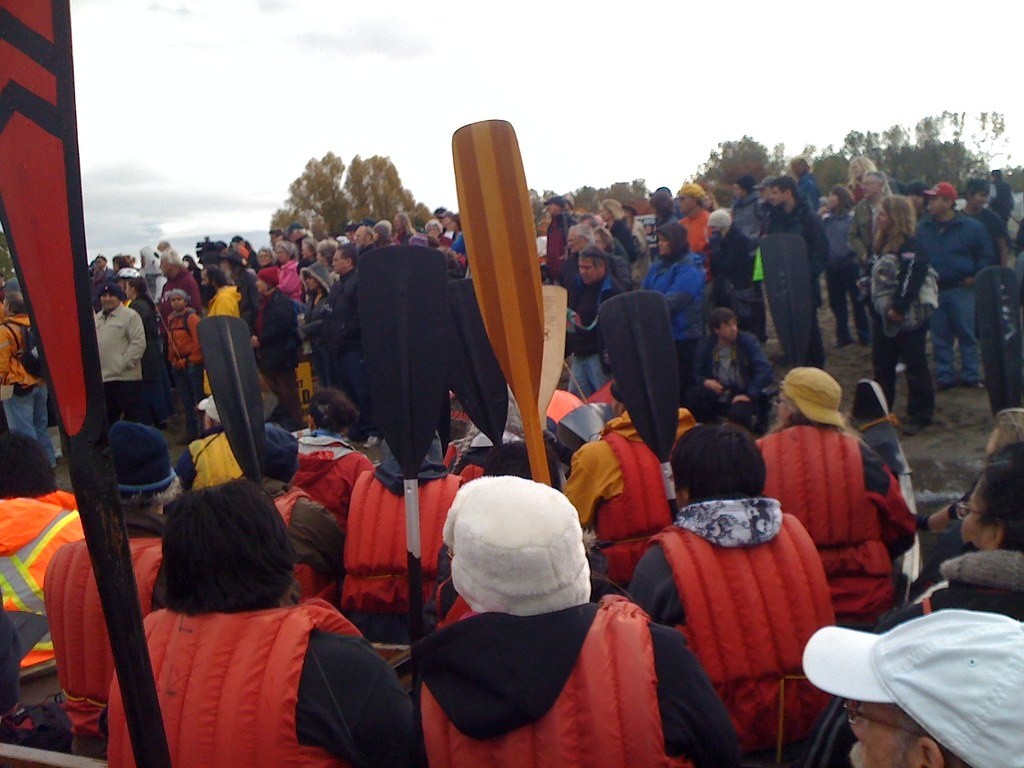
[898,414,927,434]
[932,374,957,391]
[964,378,985,391]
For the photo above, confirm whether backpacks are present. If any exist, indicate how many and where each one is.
[8,322,43,376]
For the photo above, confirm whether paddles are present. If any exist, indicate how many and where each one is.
[356,245,452,645]
[2,0,173,767]
[450,276,512,474]
[597,289,684,522]
[449,118,558,492]
[191,316,273,487]
[538,284,570,431]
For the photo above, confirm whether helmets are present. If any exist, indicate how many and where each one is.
[117,267,138,278]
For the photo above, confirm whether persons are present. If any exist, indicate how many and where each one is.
[0,154,1024,768]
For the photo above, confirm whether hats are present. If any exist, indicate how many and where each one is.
[924,182,958,198]
[260,425,299,483]
[110,423,177,492]
[440,475,592,617]
[784,366,845,428]
[374,221,391,236]
[96,285,120,300]
[707,211,728,227]
[754,175,776,189]
[544,196,566,207]
[680,183,704,201]
[287,223,300,234]
[802,607,1024,768]
[257,268,278,288]
[737,175,753,194]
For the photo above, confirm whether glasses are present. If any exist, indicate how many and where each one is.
[954,502,989,520]
[842,703,925,743]
